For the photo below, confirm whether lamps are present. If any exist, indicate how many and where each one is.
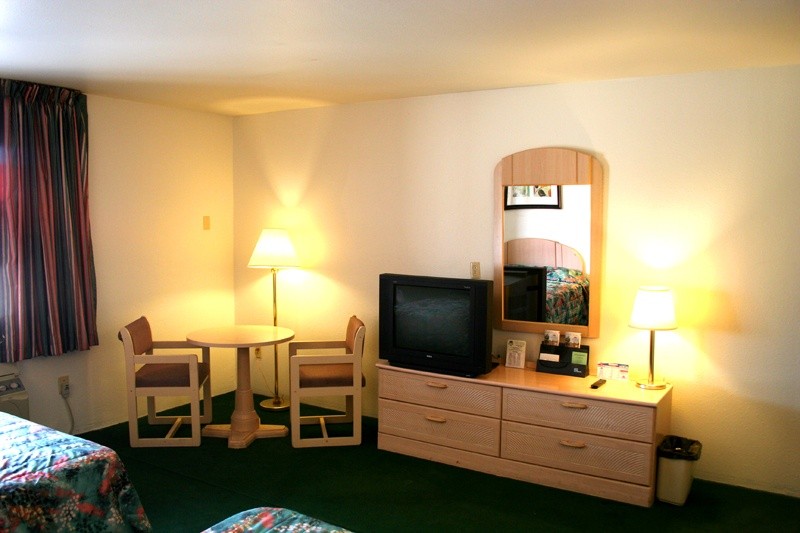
[247,229,303,411]
[627,286,677,390]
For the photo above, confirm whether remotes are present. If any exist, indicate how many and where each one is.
[591,379,606,389]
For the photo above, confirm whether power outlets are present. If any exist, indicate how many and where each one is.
[58,376,70,394]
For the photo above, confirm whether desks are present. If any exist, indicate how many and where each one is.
[185,325,296,448]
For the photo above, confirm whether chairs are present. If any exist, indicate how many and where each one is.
[289,314,366,448]
[117,316,212,447]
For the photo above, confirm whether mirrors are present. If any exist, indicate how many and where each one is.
[494,146,603,338]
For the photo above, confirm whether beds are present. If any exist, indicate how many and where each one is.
[0,410,154,533]
[505,238,590,325]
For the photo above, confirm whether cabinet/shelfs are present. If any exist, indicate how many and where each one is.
[375,362,674,507]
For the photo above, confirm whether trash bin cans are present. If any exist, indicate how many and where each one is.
[658,435,702,506]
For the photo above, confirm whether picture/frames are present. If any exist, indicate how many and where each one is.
[504,185,562,211]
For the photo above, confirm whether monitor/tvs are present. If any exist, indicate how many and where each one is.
[504,264,547,322]
[378,273,493,379]
